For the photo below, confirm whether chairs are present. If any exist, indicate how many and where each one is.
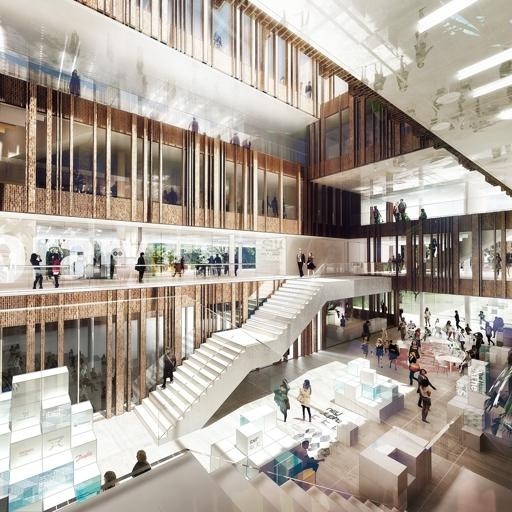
[431,347,471,376]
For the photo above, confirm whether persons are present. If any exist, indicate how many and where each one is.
[506,251,512,275]
[167,185,178,205]
[271,196,279,217]
[159,346,176,390]
[297,442,326,473]
[340,300,505,423]
[162,189,169,204]
[242,139,251,148]
[306,80,312,98]
[306,251,316,277]
[297,379,312,422]
[5,337,133,413]
[69,68,81,96]
[278,378,291,422]
[132,449,152,478]
[51,252,61,287]
[28,253,43,289]
[372,199,438,272]
[187,116,201,134]
[297,247,306,279]
[229,132,240,146]
[171,252,238,277]
[358,6,512,163]
[137,252,146,283]
[103,471,116,491]
[492,252,502,276]
[262,194,271,216]
[110,181,118,198]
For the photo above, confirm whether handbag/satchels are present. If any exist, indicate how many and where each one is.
[408,362,420,372]
